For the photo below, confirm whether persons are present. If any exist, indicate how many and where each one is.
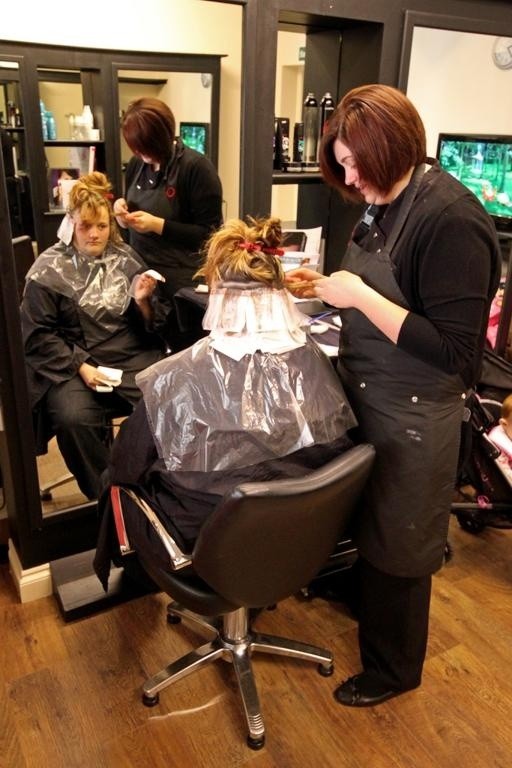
[272,231,306,252]
[48,170,82,207]
[90,213,357,593]
[277,82,500,707]
[485,392,512,489]
[18,168,181,504]
[111,95,223,359]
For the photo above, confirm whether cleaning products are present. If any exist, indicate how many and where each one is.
[41,100,56,141]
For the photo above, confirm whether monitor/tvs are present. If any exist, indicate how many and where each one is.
[434,131,512,233]
[179,121,210,157]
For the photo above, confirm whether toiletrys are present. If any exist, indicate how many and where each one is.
[293,122,304,162]
[276,117,289,162]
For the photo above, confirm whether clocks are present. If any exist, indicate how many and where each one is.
[489,36,511,67]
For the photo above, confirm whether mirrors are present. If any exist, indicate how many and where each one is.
[275,29,345,163]
[0,41,222,530]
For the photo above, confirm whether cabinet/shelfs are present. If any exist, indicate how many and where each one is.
[18,37,135,267]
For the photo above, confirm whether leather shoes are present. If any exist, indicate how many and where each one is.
[334,673,421,708]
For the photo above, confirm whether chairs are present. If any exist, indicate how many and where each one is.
[109,435,377,751]
